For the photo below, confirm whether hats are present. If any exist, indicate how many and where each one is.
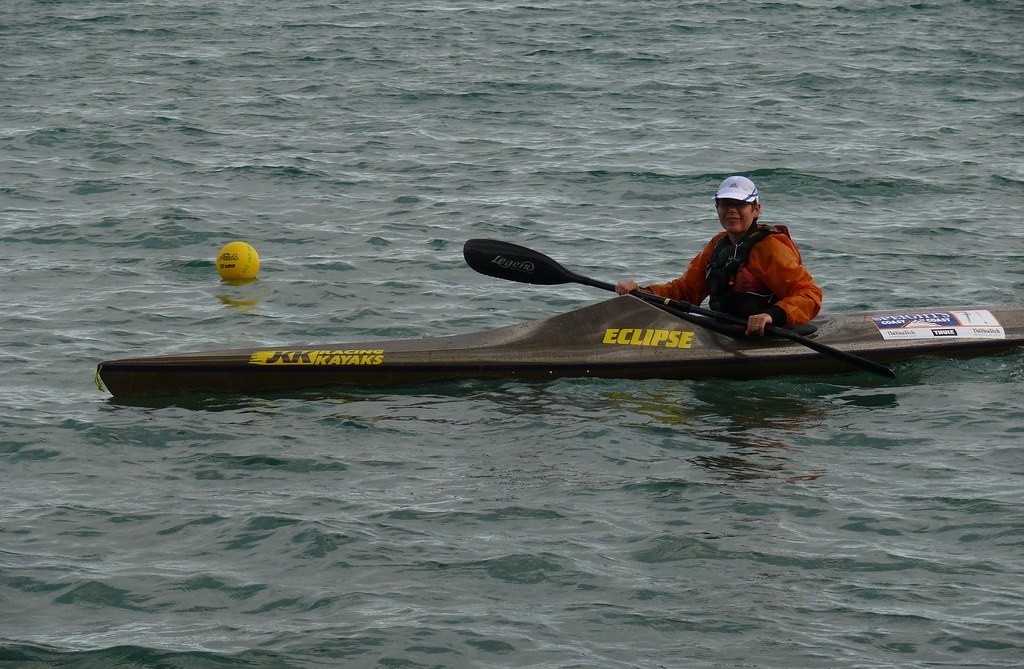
[710,176,759,204]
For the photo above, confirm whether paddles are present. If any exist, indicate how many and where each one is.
[463,237,899,379]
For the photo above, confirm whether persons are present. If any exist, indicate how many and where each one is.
[615,175,823,337]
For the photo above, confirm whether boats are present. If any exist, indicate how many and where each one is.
[93,291,1024,401]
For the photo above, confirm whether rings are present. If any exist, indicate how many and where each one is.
[755,323,760,327]
